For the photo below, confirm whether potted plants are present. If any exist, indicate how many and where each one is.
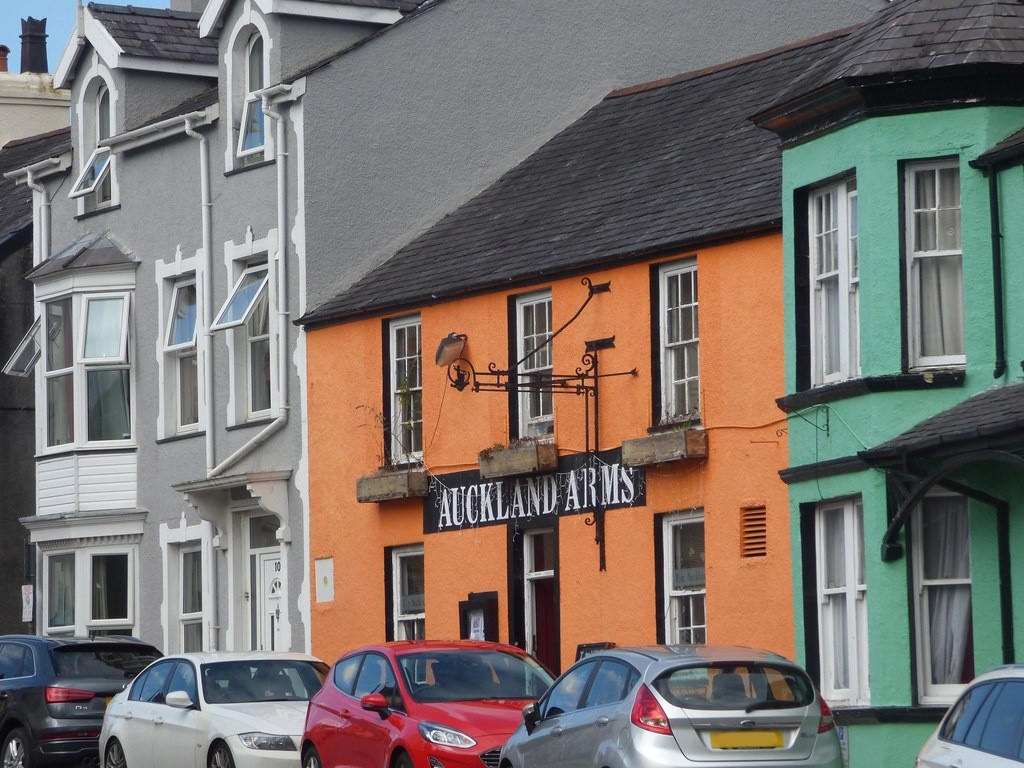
[478,433,558,479]
[356,371,428,503]
[660,444,664,444]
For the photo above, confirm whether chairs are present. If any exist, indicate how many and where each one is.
[713,673,746,700]
[459,663,501,696]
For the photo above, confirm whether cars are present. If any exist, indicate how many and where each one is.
[497,642,845,768]
[300,639,570,768]
[912,662,1024,768]
[98,651,331,768]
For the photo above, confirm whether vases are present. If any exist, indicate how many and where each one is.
[621,418,708,467]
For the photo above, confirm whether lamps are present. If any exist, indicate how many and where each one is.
[435,332,466,367]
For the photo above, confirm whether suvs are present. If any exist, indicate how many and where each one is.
[0,635,184,768]
